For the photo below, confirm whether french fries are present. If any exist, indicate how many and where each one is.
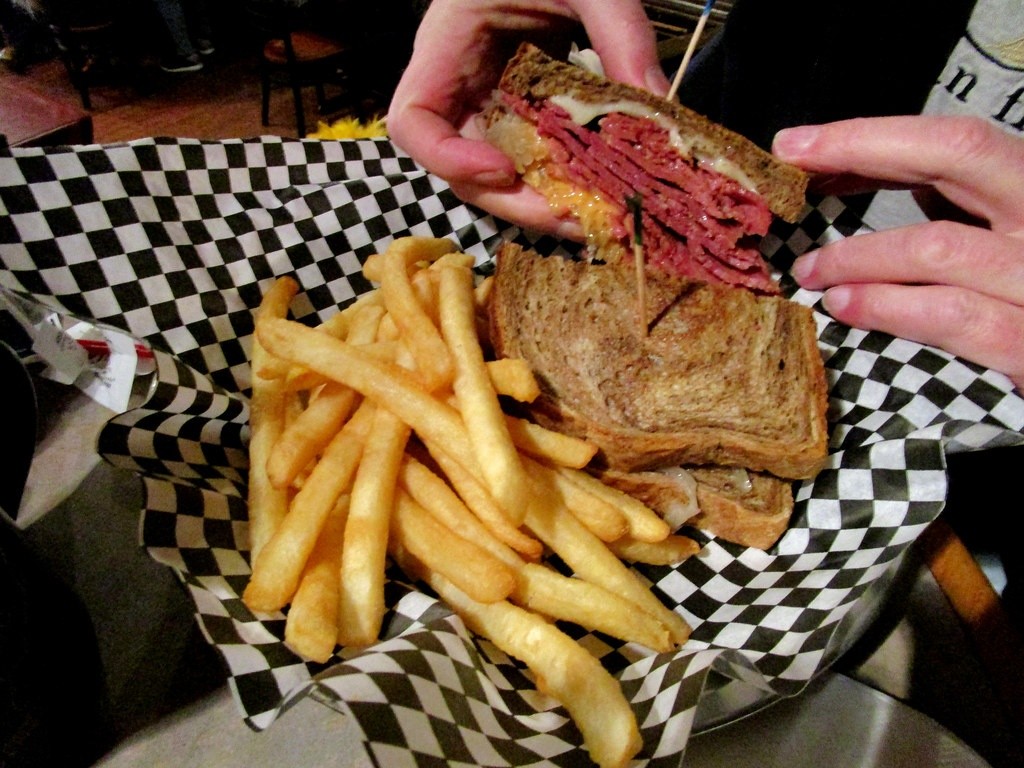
[236,233,700,767]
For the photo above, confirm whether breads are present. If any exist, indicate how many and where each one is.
[491,235,829,553]
[483,39,811,253]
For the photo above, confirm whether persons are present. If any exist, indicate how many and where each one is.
[153,0,215,73]
[386,0,1024,391]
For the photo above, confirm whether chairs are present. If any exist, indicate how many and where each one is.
[247,0,352,138]
[25,0,113,108]
